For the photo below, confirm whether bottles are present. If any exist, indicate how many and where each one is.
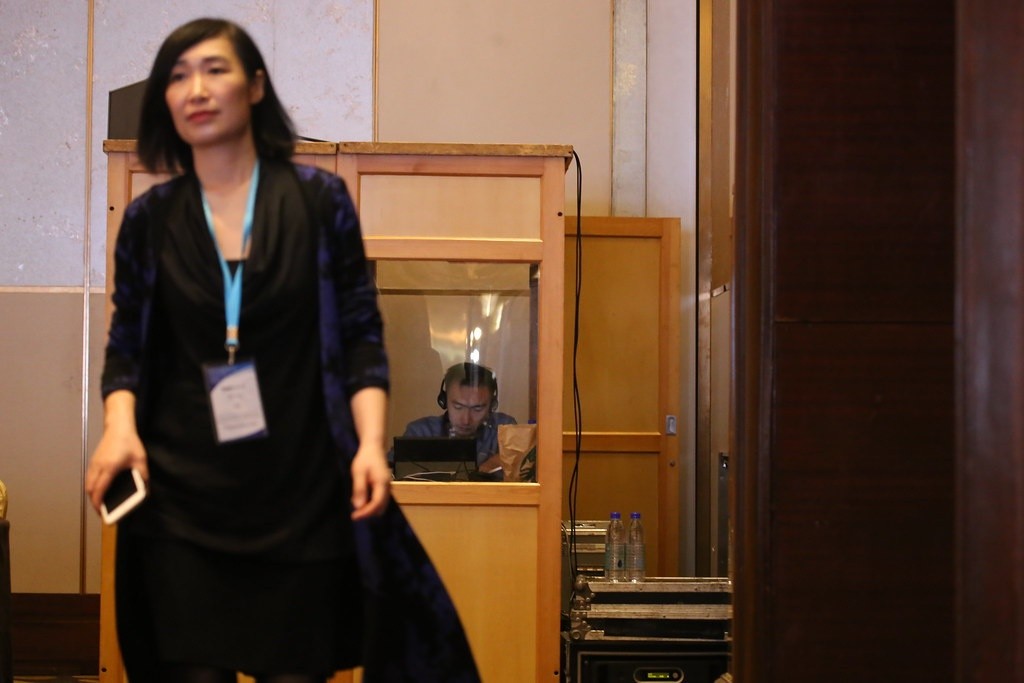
[625,512,647,583]
[604,512,627,582]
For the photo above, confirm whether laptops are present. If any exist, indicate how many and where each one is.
[393,436,479,482]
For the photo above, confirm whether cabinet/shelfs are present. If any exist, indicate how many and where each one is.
[99,139,574,683]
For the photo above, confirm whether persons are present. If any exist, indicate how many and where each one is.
[387,364,519,467]
[85,17,482,683]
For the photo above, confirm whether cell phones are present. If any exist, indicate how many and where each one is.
[99,467,147,525]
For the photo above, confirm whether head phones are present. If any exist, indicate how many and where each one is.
[438,369,498,413]
